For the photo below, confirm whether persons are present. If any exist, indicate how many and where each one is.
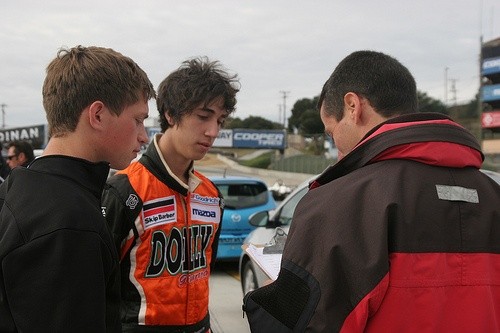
[99,56,236,333]
[244,49,499,333]
[3,139,36,172]
[0,45,156,333]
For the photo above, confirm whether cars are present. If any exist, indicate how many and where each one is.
[201,176,277,262]
[239,175,321,300]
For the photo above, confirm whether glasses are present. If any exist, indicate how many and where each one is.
[6,155,17,160]
